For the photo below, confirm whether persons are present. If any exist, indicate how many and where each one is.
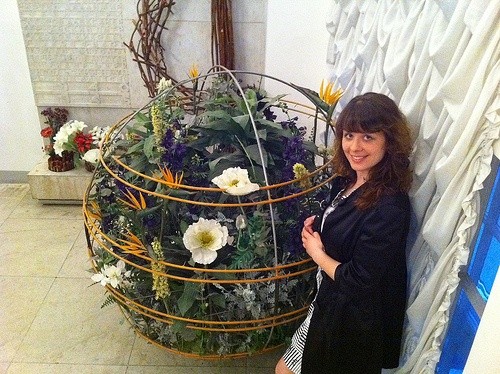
[275,91,414,374]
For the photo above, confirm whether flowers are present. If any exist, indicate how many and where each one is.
[41,108,123,165]
[87,65,345,355]
